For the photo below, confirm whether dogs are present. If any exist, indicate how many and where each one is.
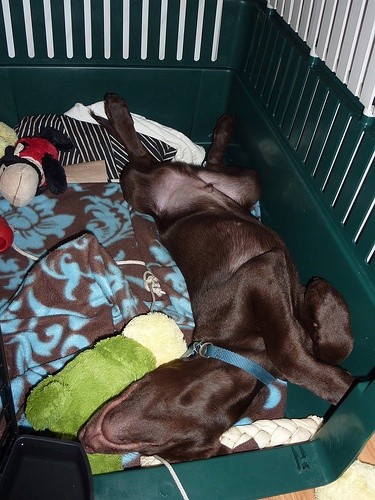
[76,92,361,464]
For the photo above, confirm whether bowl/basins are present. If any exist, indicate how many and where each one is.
[1,435,93,500]
